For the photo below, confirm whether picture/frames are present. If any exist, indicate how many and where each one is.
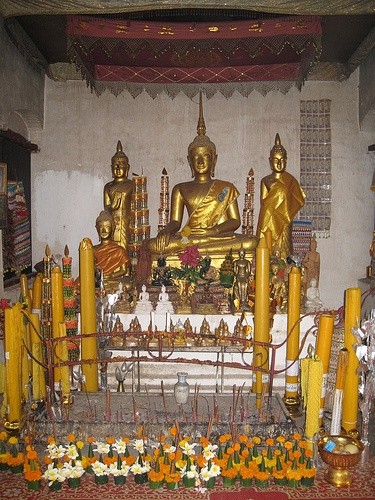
[0,163,9,229]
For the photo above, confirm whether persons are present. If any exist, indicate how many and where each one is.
[233,242,251,308]
[152,256,174,286]
[197,255,220,284]
[148,135,259,255]
[137,284,152,304]
[112,282,138,310]
[111,315,246,343]
[269,262,286,313]
[302,232,320,304]
[157,285,172,305]
[269,247,286,282]
[104,140,133,277]
[75,210,133,292]
[256,133,306,260]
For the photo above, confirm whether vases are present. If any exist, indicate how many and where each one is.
[174,372,189,405]
[177,279,190,307]
[0,462,315,490]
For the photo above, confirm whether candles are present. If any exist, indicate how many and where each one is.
[253,238,361,458]
[0,238,97,433]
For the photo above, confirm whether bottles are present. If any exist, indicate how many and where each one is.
[173,372,189,405]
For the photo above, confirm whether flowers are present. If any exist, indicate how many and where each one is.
[0,433,317,486]
[169,245,203,281]
